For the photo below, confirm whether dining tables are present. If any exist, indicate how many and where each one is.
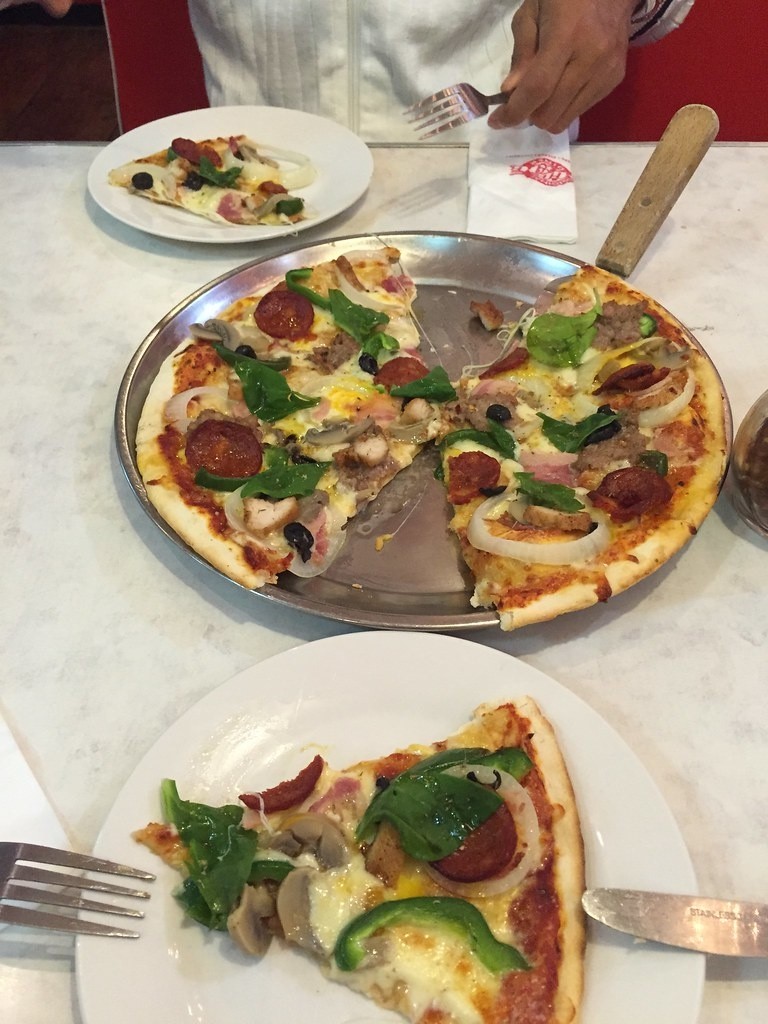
[0,136,768,1024]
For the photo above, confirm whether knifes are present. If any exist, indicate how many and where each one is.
[578,885,768,960]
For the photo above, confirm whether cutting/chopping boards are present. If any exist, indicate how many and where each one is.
[109,230,736,630]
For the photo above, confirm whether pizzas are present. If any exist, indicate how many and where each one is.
[133,694,587,1024]
[134,247,732,629]
[107,133,314,226]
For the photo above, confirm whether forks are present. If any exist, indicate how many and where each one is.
[398,81,510,142]
[0,841,157,940]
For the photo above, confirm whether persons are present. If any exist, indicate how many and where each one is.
[0,0,699,151]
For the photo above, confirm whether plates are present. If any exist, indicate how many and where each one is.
[87,104,376,244]
[66,630,704,1024]
